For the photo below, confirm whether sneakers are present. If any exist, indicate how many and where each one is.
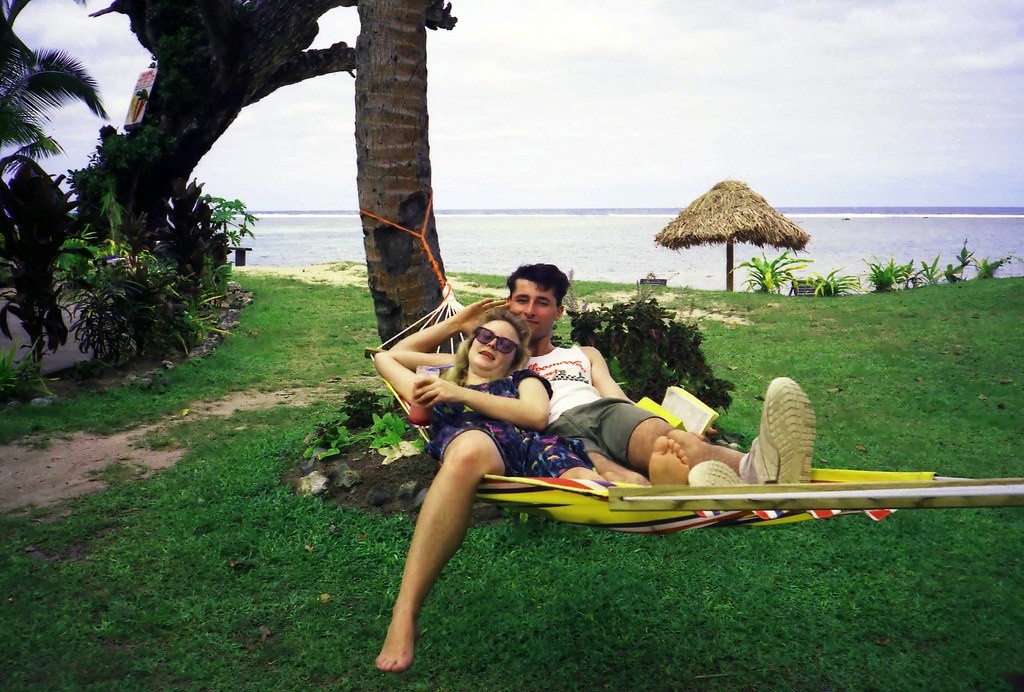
[687,461,741,487]
[750,376,817,484]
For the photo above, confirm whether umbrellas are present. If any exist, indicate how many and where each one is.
[654,180,810,290]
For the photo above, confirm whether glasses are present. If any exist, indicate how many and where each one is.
[475,326,519,354]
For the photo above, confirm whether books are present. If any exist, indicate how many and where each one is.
[638,387,719,435]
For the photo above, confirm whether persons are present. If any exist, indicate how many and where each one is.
[374,311,690,673]
[389,264,815,485]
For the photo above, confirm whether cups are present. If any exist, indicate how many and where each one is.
[408,366,440,429]
